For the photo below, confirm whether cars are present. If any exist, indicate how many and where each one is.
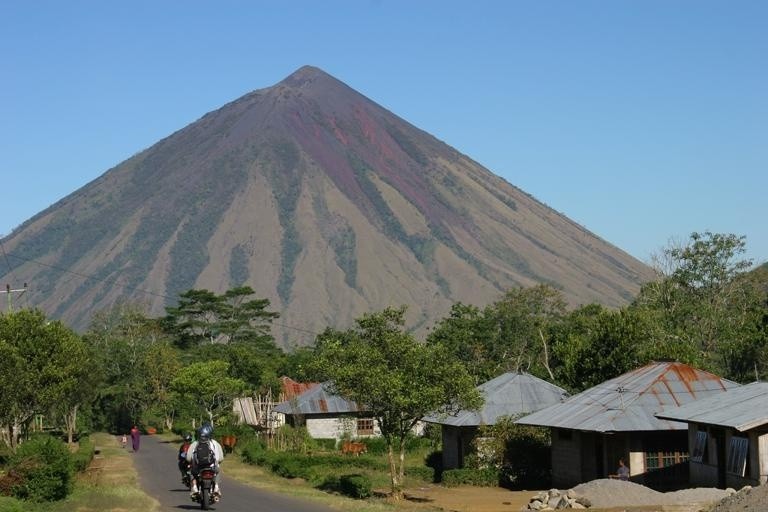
[499,463,538,491]
[49,431,81,442]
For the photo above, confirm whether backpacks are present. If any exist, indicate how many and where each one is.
[192,441,216,467]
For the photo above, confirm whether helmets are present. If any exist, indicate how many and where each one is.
[196,423,214,437]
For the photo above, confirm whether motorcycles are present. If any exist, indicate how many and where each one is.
[181,462,220,510]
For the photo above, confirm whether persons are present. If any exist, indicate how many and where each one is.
[119,434,128,450]
[187,423,225,499]
[177,432,195,489]
[130,424,140,452]
[609,459,630,481]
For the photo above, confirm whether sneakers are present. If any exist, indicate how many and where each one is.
[214,487,222,498]
[189,487,199,496]
